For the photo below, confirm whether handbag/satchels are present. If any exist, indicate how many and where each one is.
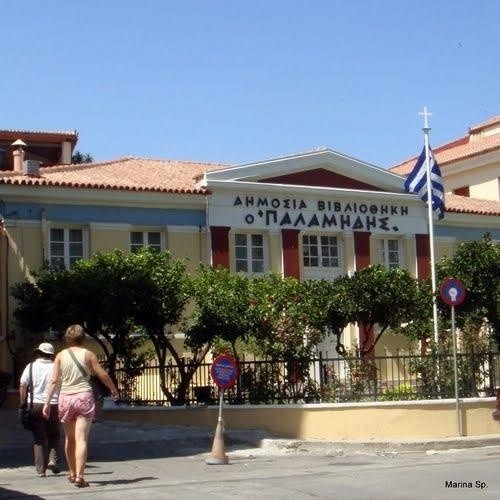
[16,402,32,430]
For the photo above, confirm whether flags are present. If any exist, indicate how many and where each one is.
[404,141,444,220]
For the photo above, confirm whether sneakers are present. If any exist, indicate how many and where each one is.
[36,473,46,478]
[47,461,62,474]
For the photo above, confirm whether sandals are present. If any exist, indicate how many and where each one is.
[73,477,89,487]
[66,475,76,483]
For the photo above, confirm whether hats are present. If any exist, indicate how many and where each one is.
[33,342,55,355]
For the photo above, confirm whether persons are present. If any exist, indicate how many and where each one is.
[20,342,62,477]
[42,324,120,488]
